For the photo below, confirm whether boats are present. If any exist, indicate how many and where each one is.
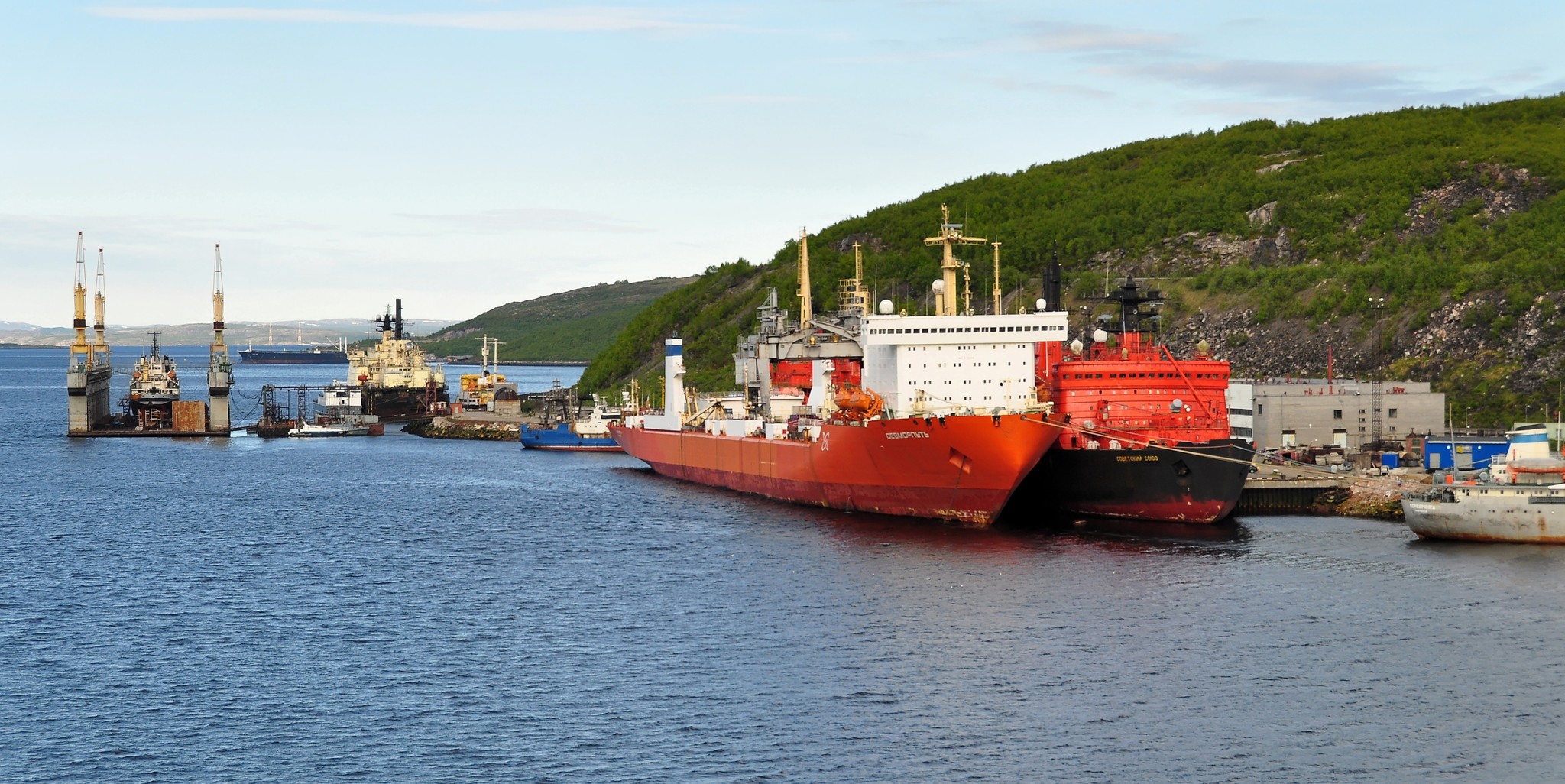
[168,371,176,377]
[518,377,649,451]
[606,204,1256,535]
[849,388,870,411]
[132,372,140,378]
[1400,497,1565,543]
[288,420,370,437]
[834,387,852,410]
[334,299,451,417]
[455,334,518,410]
[129,328,180,422]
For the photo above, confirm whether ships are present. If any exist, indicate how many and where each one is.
[237,337,350,364]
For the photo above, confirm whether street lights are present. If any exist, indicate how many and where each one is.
[1466,407,1471,433]
[1526,405,1530,423]
